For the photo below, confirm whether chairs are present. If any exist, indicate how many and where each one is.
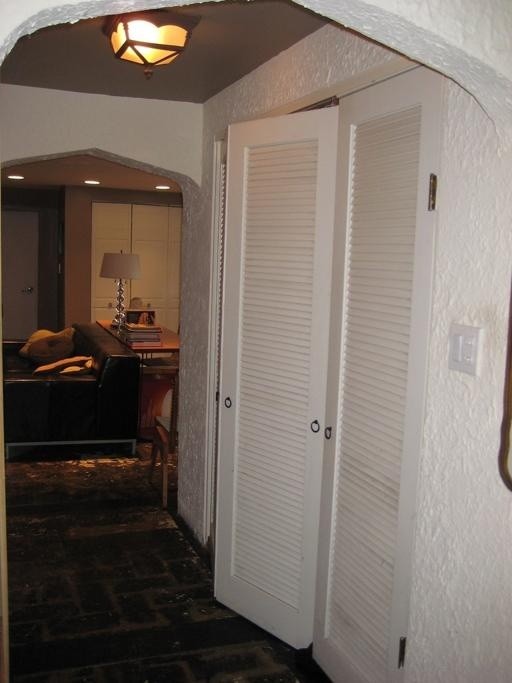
[149,369,178,510]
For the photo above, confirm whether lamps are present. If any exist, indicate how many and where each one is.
[100,248,141,329]
[101,5,202,80]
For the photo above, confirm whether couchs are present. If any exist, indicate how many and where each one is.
[3,322,141,464]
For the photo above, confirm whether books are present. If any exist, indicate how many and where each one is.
[126,324,162,348]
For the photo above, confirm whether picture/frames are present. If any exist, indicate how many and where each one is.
[497,270,512,493]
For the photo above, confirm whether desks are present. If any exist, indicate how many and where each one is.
[96,319,180,441]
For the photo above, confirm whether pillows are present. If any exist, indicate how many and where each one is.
[58,358,101,379]
[31,355,93,377]
[18,326,55,358]
[28,327,76,367]
